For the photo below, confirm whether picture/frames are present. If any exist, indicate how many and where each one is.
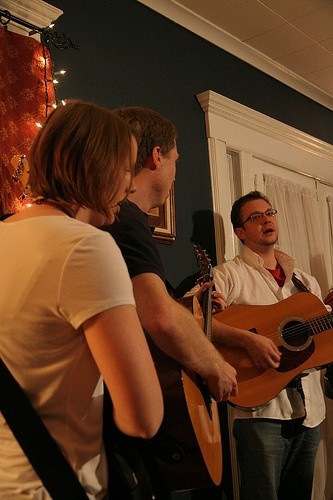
[146,183,176,244]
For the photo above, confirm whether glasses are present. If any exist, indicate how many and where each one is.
[239,209,277,227]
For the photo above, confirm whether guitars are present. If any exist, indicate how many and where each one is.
[211,291,333,413]
[180,244,224,488]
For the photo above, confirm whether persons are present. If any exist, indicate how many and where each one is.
[210,190,333,500]
[104,107,240,500]
[3,102,164,499]
